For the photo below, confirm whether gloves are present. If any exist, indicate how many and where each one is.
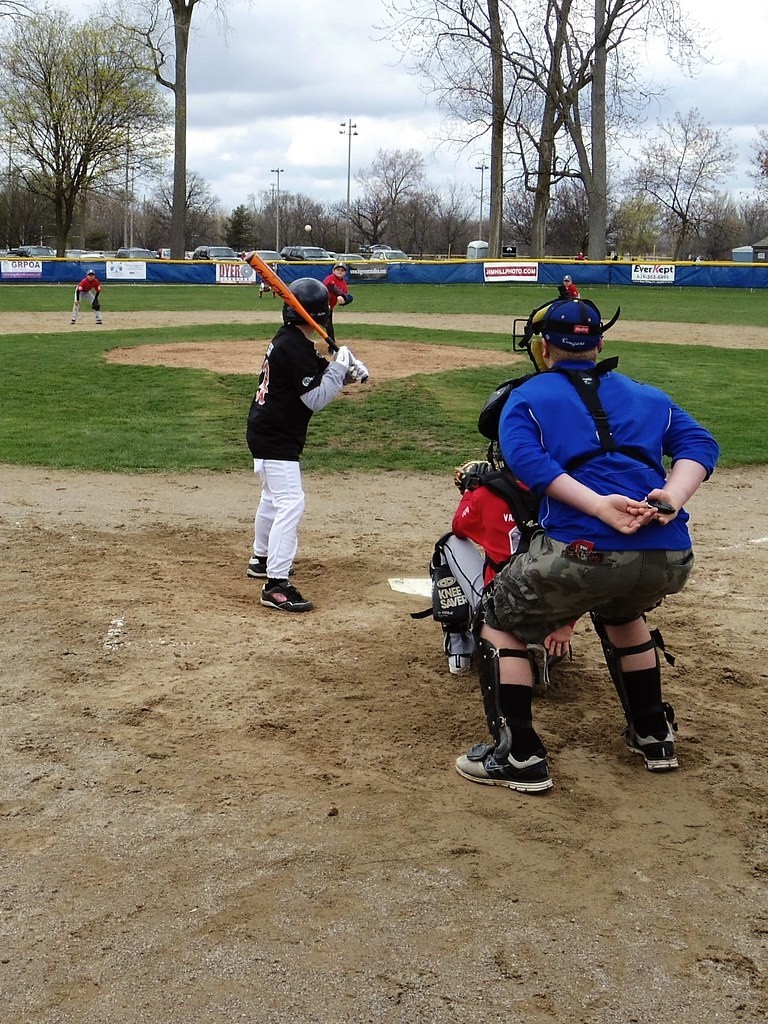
[332,345,368,380]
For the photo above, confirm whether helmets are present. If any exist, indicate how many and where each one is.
[282,277,329,325]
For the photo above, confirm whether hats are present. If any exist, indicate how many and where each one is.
[563,275,572,282]
[542,300,602,351]
[334,263,347,271]
[87,269,95,275]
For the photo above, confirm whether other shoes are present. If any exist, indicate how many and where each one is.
[328,346,334,354]
[260,295,262,298]
[70,320,75,324]
[96,320,102,324]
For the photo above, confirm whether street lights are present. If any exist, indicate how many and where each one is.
[475,164,489,240]
[270,167,284,253]
[339,118,358,254]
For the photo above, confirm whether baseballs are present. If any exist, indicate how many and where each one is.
[305,224,312,232]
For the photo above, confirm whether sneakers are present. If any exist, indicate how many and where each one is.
[246,555,295,578]
[625,720,679,772]
[455,749,553,792]
[448,654,470,674]
[260,582,313,612]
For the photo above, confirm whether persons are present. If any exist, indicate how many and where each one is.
[72,269,103,324]
[430,439,579,676]
[322,263,354,354]
[258,275,265,298]
[457,298,720,792]
[245,277,369,612]
[562,275,581,300]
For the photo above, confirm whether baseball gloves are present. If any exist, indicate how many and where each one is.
[339,293,353,306]
[91,298,101,311]
[454,461,493,496]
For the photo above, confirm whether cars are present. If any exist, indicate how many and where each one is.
[0,244,414,260]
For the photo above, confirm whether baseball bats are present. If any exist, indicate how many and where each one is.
[244,250,369,383]
